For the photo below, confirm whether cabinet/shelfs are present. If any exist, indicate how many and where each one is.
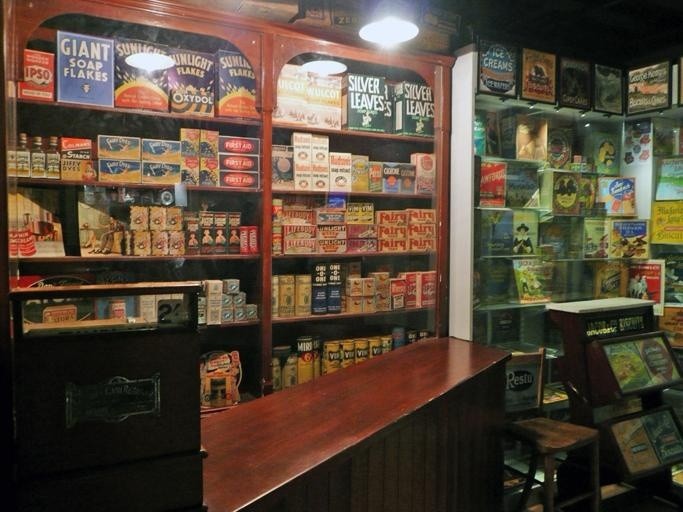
[265,19,449,393]
[4,0,268,424]
[449,52,681,423]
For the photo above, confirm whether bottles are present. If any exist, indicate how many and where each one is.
[44,136,61,179]
[108,300,127,321]
[14,130,30,177]
[29,136,47,179]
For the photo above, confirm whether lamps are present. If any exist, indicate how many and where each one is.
[352,3,429,45]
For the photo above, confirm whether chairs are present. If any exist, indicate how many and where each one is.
[495,345,596,507]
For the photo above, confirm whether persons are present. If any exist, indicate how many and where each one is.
[97,215,125,254]
[627,270,640,298]
[512,223,533,254]
